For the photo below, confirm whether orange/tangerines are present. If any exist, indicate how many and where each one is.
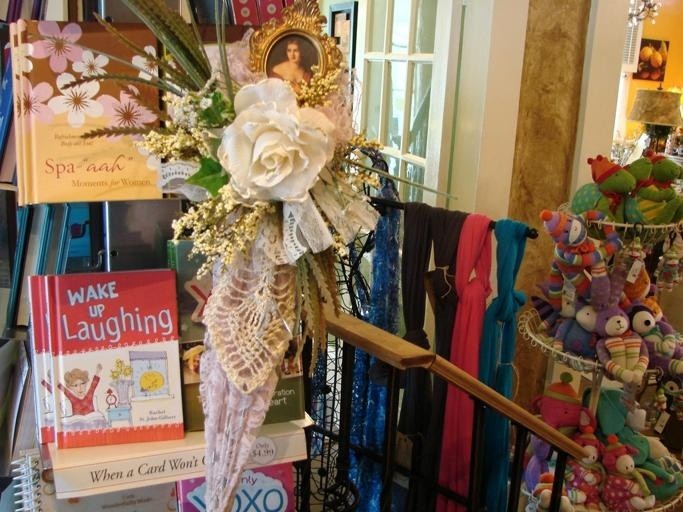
[639,40,668,80]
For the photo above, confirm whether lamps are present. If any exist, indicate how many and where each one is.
[626,88,683,152]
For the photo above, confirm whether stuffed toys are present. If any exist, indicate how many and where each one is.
[520,147,682,512]
[519,148,683,512]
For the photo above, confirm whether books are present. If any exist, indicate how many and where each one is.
[0,1,312,511]
